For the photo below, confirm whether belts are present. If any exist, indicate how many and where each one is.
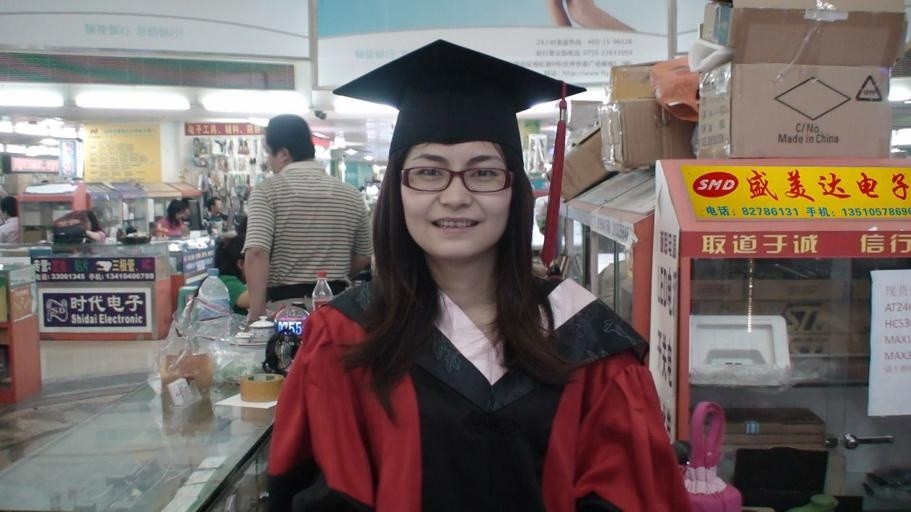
[269,281,350,300]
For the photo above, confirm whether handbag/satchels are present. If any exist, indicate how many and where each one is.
[679,399,743,512]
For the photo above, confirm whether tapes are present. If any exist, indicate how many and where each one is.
[240,373,284,402]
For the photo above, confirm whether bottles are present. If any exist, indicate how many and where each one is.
[312,271,334,312]
[197,268,230,390]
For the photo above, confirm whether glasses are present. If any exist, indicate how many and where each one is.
[400,161,512,194]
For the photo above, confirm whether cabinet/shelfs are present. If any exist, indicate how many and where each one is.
[561,157,911,512]
[0,181,216,402]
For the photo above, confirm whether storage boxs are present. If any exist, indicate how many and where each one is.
[546,127,611,202]
[605,56,692,103]
[594,100,697,175]
[695,2,909,158]
[568,101,604,131]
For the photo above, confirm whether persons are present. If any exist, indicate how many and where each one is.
[237,113,375,334]
[151,175,382,319]
[265,94,693,512]
[83,209,106,244]
[0,195,20,246]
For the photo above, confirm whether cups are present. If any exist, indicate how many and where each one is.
[235,315,275,345]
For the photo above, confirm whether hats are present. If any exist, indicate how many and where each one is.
[331,38,589,152]
